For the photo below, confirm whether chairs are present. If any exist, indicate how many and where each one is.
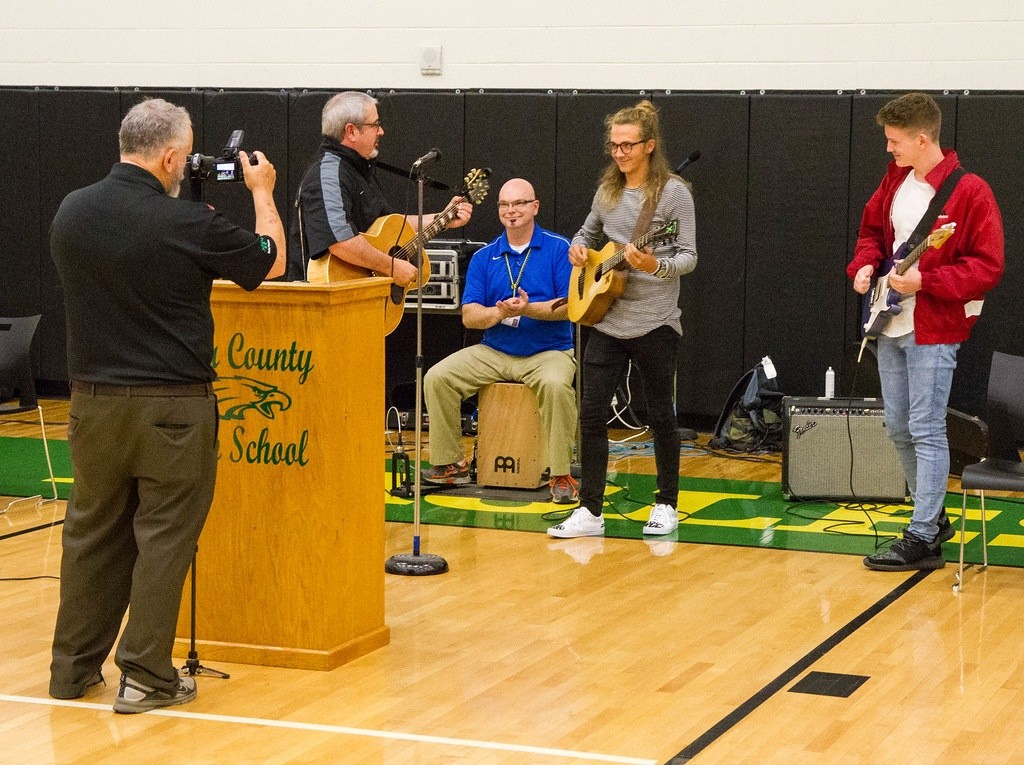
[0,313,58,515]
[952,351,1024,592]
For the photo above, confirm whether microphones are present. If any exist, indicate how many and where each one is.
[412,147,443,167]
[675,148,702,175]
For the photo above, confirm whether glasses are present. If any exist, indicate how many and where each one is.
[364,119,382,130]
[605,139,644,154]
[498,199,532,209]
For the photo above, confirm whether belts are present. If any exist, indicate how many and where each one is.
[70,381,212,396]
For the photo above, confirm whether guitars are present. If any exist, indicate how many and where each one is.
[861,222,957,341]
[567,216,680,328]
[306,169,490,338]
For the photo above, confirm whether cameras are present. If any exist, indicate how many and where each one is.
[212,130,258,183]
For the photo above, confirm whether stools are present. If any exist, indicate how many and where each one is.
[476,382,551,492]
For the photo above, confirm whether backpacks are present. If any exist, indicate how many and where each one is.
[707,361,787,454]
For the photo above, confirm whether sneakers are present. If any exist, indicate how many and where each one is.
[77,666,106,698]
[113,673,197,714]
[937,507,956,542]
[547,509,605,538]
[642,504,679,534]
[420,462,471,484]
[549,475,579,504]
[864,529,946,573]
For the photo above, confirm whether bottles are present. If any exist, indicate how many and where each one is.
[825,366,835,398]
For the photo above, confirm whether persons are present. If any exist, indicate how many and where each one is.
[845,93,1004,572]
[288,91,473,288]
[421,177,579,504]
[546,99,698,538]
[49,97,287,713]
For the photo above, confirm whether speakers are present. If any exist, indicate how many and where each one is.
[781,395,907,503]
